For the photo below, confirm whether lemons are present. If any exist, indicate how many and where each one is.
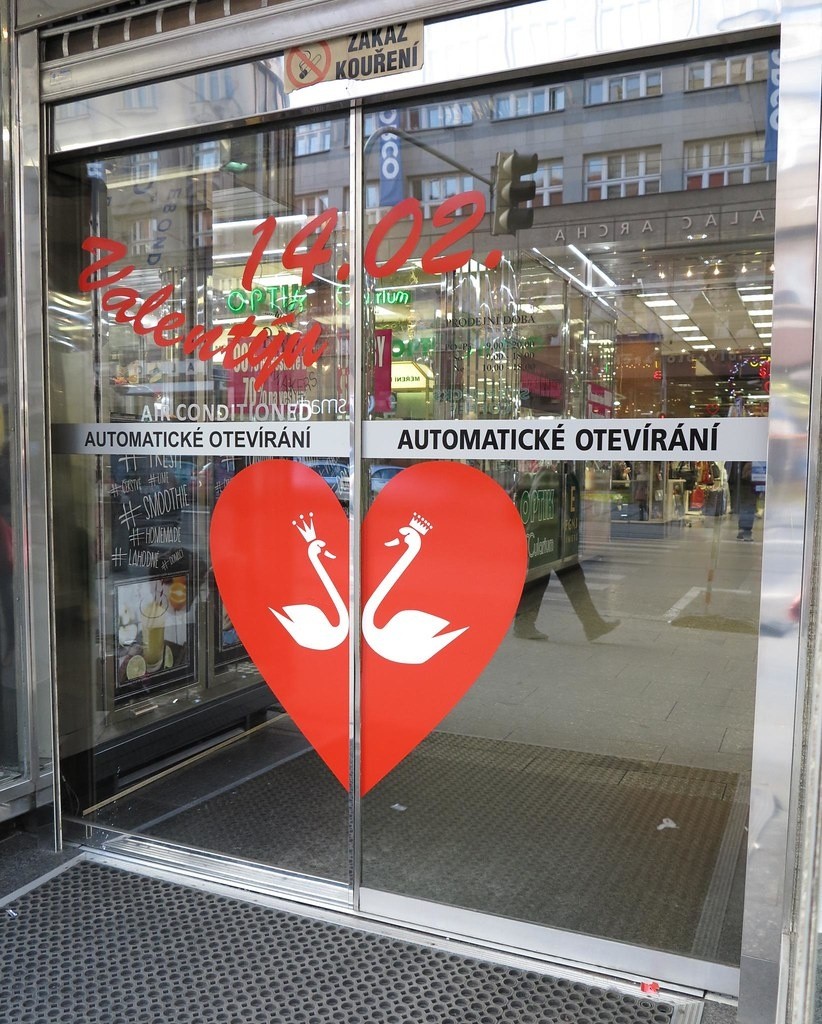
[164,645,173,668]
[126,655,147,680]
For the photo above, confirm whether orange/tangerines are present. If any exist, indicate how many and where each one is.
[168,582,186,608]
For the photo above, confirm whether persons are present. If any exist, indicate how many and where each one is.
[511,456,622,644]
[722,405,764,542]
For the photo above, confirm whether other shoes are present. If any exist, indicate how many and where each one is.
[513,628,548,639]
[587,619,621,641]
[737,531,754,541]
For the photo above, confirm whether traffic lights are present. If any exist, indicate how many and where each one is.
[490,147,539,236]
